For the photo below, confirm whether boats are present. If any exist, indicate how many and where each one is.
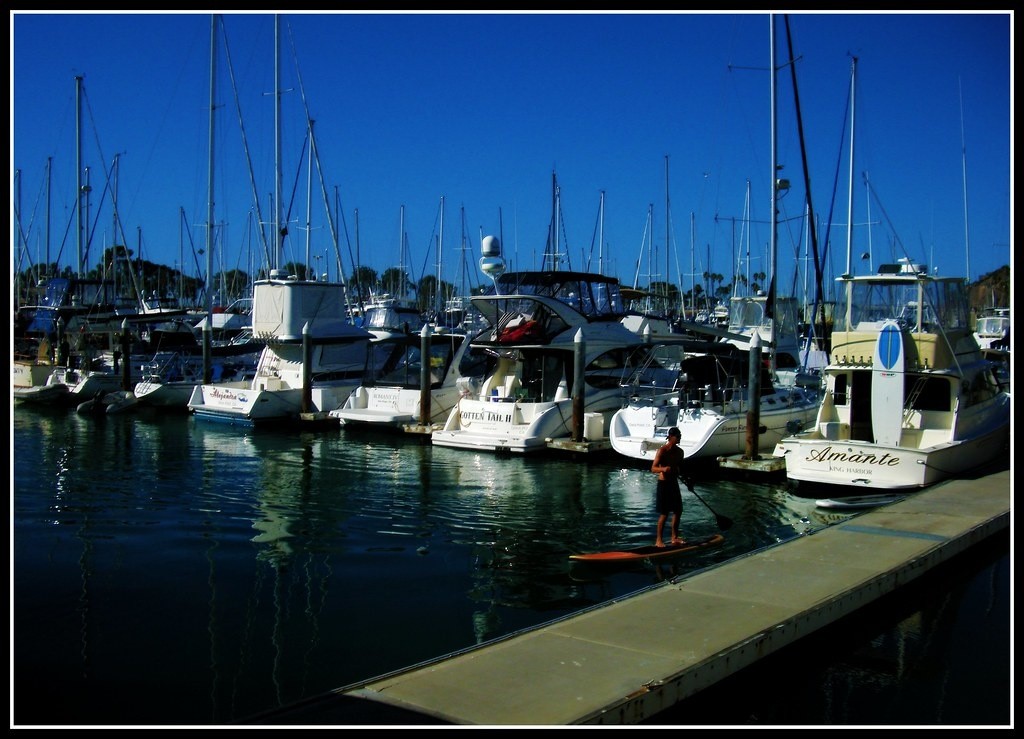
[816,497,907,512]
[564,534,729,561]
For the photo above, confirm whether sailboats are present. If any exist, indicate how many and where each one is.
[11,11,1010,496]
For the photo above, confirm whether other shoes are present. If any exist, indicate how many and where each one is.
[671,538,686,544]
[656,541,665,547]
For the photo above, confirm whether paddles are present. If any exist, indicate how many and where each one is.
[676,470,734,531]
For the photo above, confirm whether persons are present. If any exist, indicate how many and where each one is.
[652,427,686,548]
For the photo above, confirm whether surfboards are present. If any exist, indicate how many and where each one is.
[569,534,723,561]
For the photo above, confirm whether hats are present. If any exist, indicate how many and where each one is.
[666,428,681,440]
